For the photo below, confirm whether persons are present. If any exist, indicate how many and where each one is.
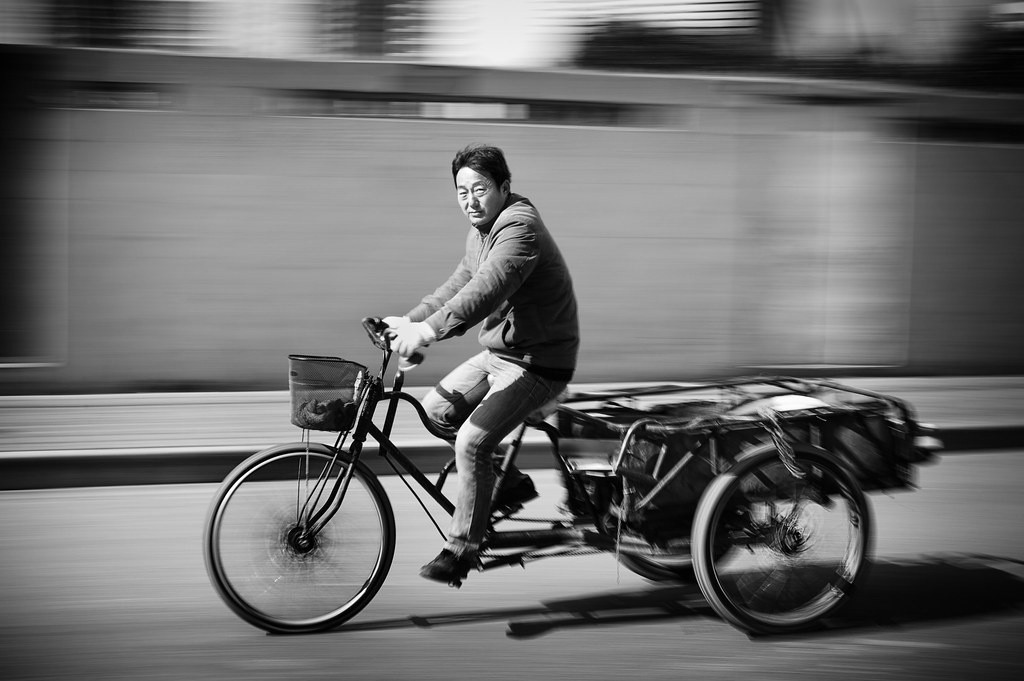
[378,145,579,582]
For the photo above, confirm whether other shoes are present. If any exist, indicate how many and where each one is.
[490,474,538,510]
[421,549,475,584]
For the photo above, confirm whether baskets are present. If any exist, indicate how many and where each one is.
[288,354,368,431]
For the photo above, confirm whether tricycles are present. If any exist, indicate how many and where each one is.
[201,316,947,637]
